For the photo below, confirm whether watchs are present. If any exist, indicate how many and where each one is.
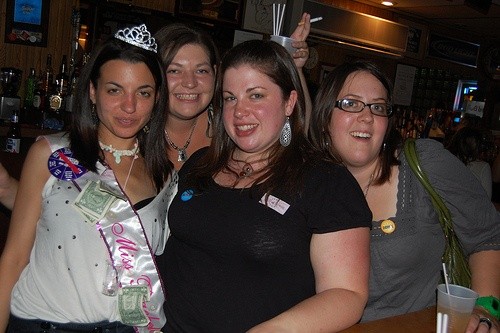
[476,296,500,319]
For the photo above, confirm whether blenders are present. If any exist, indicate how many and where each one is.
[1,68,20,123]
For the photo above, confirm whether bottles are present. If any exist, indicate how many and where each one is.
[5,109,23,163]
[23,51,81,118]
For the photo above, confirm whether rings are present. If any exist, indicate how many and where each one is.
[480,317,494,330]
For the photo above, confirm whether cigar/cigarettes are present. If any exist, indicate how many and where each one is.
[298,16,324,25]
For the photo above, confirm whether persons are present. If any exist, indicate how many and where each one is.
[306,59,500,333]
[152,39,374,333]
[1,13,311,214]
[390,98,500,209]
[1,23,182,333]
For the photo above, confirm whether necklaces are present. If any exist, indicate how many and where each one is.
[225,151,279,178]
[124,144,140,195]
[98,136,140,164]
[365,158,379,198]
[164,117,201,163]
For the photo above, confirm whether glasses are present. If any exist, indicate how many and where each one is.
[336,98,395,119]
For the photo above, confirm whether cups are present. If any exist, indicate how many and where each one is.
[434,284,477,332]
